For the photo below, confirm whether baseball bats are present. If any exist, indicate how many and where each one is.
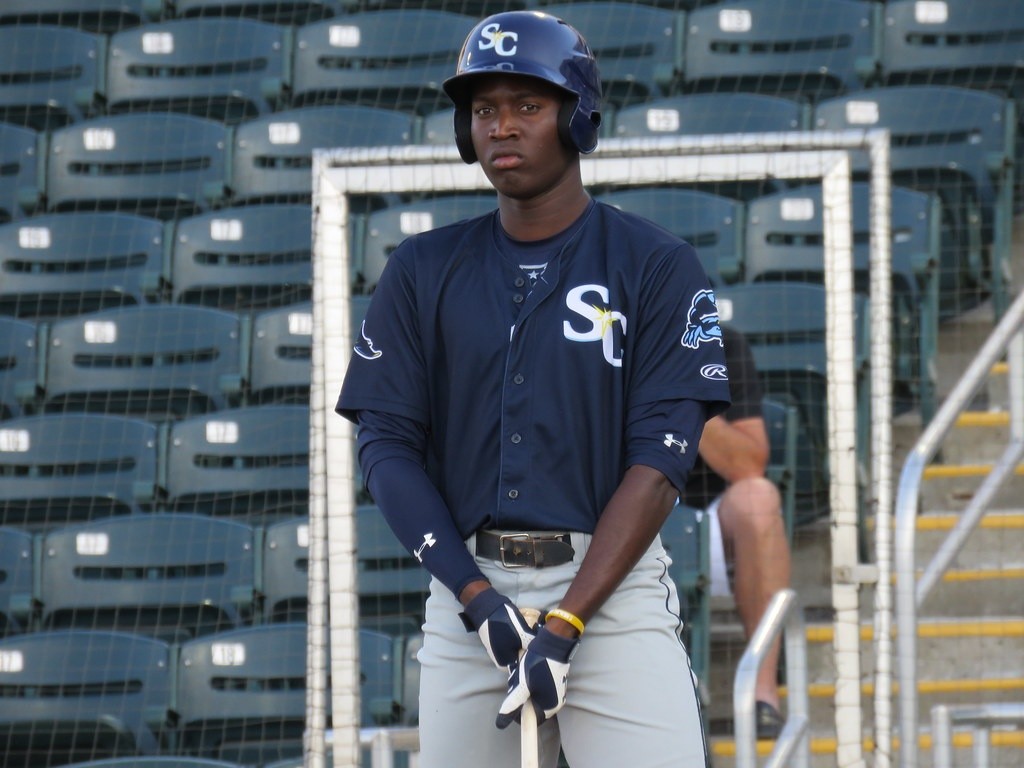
[519,607,542,768]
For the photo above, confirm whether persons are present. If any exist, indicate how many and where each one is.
[335,12,733,768]
[679,326,789,740]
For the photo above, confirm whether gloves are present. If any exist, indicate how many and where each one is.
[496,626,583,730]
[457,586,537,675]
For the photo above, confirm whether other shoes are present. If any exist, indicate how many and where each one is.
[755,700,783,738]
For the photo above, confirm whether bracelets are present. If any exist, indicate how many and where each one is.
[545,609,585,638]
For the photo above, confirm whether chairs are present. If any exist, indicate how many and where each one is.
[0,0,1024,768]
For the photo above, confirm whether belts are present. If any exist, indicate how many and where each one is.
[475,529,575,570]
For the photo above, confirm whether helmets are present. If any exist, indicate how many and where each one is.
[442,9,603,165]
